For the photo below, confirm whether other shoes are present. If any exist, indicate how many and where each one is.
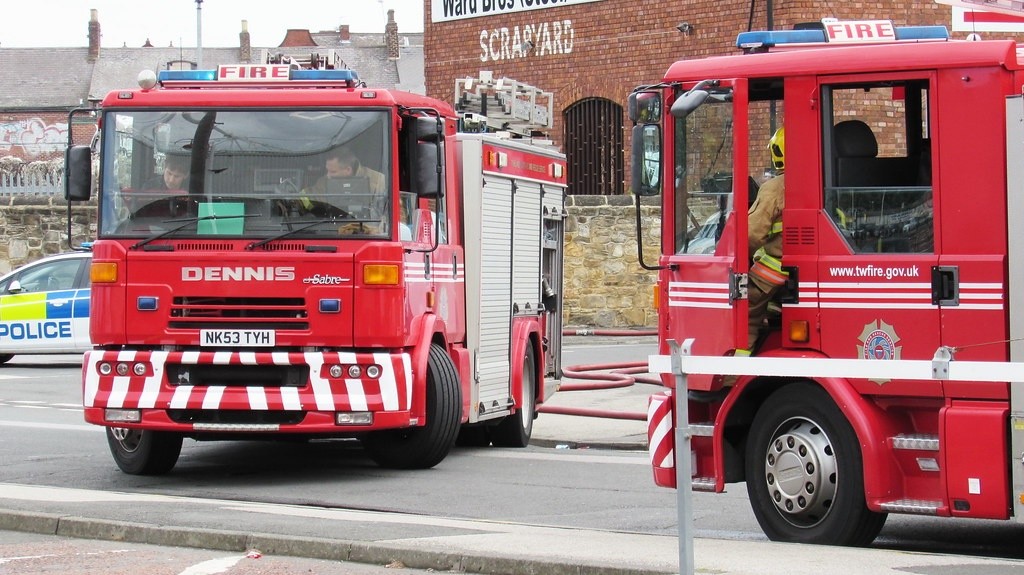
[686,385,733,402]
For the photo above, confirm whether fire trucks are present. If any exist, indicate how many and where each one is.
[63,50,568,470]
[628,0,1024,548]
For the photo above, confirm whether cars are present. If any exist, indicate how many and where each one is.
[0,240,92,364]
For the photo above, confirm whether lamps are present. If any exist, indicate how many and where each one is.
[676,22,693,33]
[525,40,534,50]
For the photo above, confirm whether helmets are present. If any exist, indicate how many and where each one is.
[767,125,785,169]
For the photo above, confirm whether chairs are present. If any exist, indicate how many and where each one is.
[834,120,882,205]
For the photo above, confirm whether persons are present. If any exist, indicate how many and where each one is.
[296,146,406,233]
[638,108,650,123]
[131,159,191,214]
[688,124,790,404]
